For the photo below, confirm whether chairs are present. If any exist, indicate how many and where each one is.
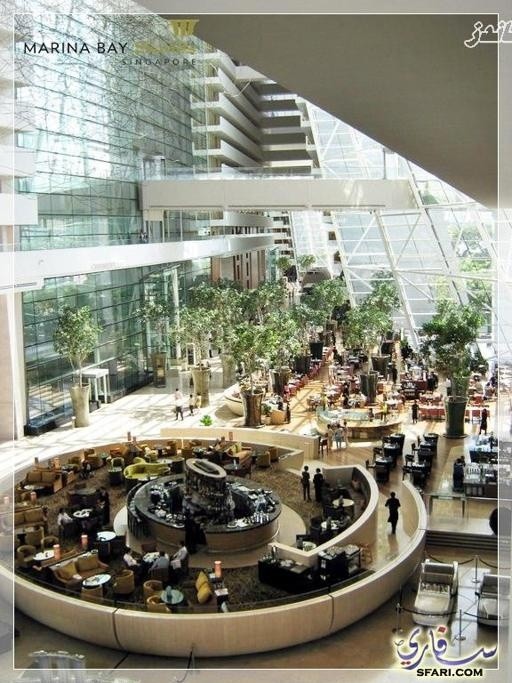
[1,438,361,613]
[363,430,425,480]
[417,403,490,422]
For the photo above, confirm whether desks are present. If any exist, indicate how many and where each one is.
[412,431,440,487]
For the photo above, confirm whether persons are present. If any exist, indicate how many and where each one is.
[453,456,466,492]
[342,383,349,409]
[174,388,201,421]
[302,466,311,501]
[183,510,198,555]
[391,365,397,384]
[478,409,488,436]
[313,468,322,503]
[57,508,74,527]
[96,486,108,529]
[384,492,400,535]
[411,400,419,424]
[122,540,188,586]
[445,378,451,396]
[82,460,96,479]
[429,372,438,394]
[326,422,349,452]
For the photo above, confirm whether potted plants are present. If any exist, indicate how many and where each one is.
[50,302,105,428]
[137,269,485,439]
